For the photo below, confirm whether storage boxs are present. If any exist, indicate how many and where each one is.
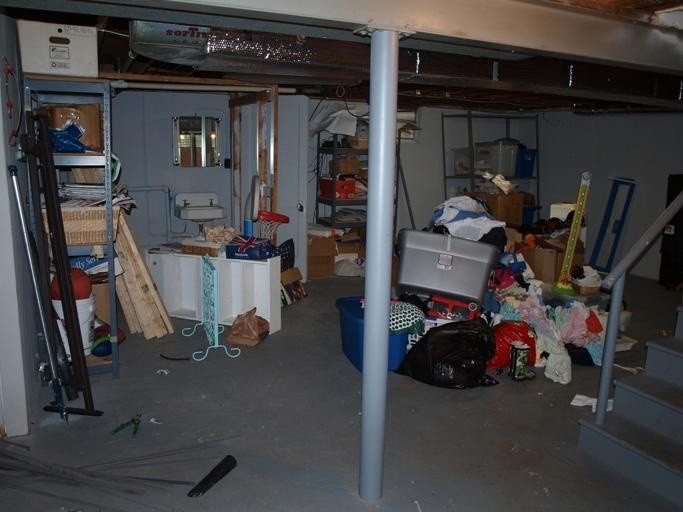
[453,143,518,177]
[307,238,336,280]
[329,156,360,177]
[516,245,585,288]
[18,19,99,80]
[318,175,355,200]
[37,103,102,152]
[91,282,111,330]
[467,192,524,227]
[394,227,497,312]
[515,150,537,177]
[523,206,536,225]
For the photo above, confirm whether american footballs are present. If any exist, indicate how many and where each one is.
[93,326,126,344]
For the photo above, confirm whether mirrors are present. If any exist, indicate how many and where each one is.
[173,116,221,168]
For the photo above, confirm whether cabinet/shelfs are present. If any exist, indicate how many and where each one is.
[314,125,398,259]
[144,244,281,337]
[442,110,542,228]
[23,81,128,379]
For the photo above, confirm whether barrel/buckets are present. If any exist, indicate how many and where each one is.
[51,292,96,362]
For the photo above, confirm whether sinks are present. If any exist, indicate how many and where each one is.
[180,205,222,212]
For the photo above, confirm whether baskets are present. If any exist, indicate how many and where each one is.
[41,205,122,245]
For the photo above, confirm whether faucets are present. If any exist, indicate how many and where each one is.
[182,199,191,208]
[208,198,215,208]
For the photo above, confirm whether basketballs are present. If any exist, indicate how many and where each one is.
[51,269,92,300]
